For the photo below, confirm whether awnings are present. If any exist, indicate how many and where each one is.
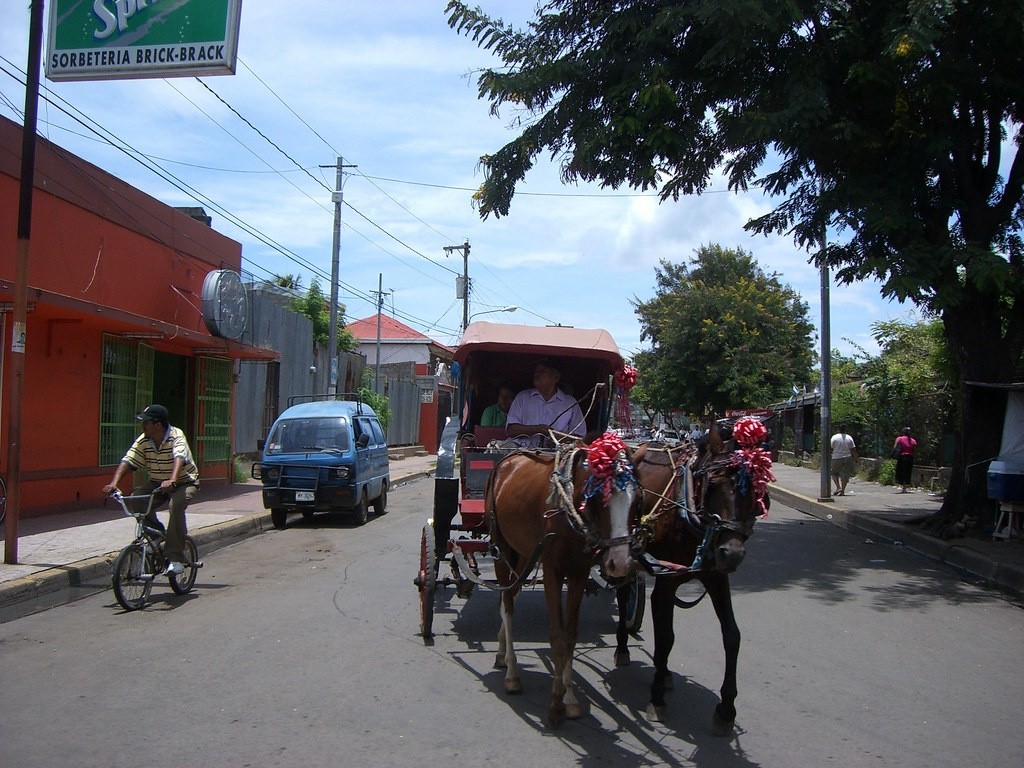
[720,412,776,429]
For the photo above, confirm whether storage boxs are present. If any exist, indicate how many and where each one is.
[986,460,1024,501]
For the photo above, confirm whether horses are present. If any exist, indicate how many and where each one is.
[614,412,770,738]
[484,431,647,729]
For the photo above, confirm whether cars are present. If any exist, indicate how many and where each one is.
[616,428,650,440]
[653,429,682,448]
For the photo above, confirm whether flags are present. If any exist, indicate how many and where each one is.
[792,384,799,397]
[813,385,821,395]
[802,382,806,396]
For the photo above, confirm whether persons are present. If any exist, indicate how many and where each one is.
[893,427,917,494]
[657,429,666,442]
[102,404,202,576]
[691,425,702,441]
[480,381,524,427]
[830,422,859,496]
[648,427,657,440]
[680,427,691,443]
[505,359,587,445]
[554,366,603,426]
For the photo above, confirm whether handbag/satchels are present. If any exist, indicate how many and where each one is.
[892,438,901,460]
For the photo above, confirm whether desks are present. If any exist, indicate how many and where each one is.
[992,501,1024,541]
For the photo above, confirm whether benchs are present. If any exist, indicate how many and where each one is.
[460,424,508,460]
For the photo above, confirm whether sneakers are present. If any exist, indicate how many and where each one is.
[146,537,162,554]
[165,562,184,574]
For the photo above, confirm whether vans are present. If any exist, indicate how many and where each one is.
[252,393,390,530]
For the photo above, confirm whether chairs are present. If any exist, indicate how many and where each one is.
[335,432,349,451]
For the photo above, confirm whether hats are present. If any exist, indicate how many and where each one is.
[135,404,168,421]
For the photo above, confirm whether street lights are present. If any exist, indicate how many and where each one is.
[463,305,518,333]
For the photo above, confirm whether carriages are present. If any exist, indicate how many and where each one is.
[414,321,777,736]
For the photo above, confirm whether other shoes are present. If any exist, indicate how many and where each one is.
[839,493,844,496]
[834,489,842,495]
[901,490,907,493]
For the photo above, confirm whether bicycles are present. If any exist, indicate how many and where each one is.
[103,482,203,611]
